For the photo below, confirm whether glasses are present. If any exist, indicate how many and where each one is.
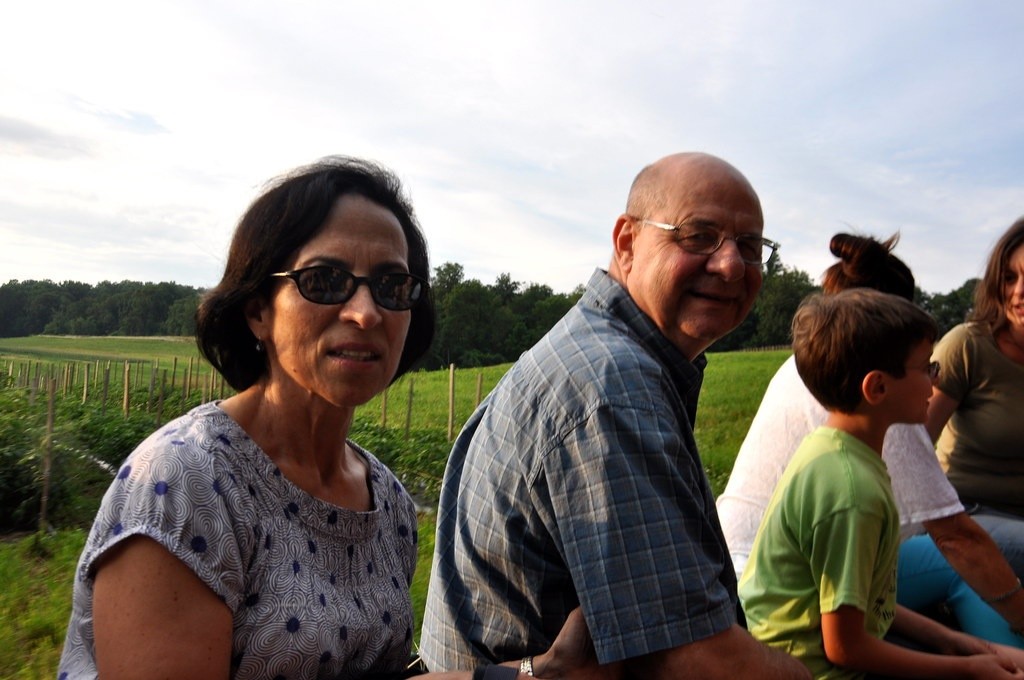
[270,264,430,311]
[906,361,942,385]
[631,217,779,265]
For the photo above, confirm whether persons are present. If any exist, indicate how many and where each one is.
[419,152,816,680]
[59,155,622,680]
[715,219,1024,680]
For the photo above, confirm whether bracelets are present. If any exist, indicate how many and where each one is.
[981,576,1023,602]
[520,656,534,677]
